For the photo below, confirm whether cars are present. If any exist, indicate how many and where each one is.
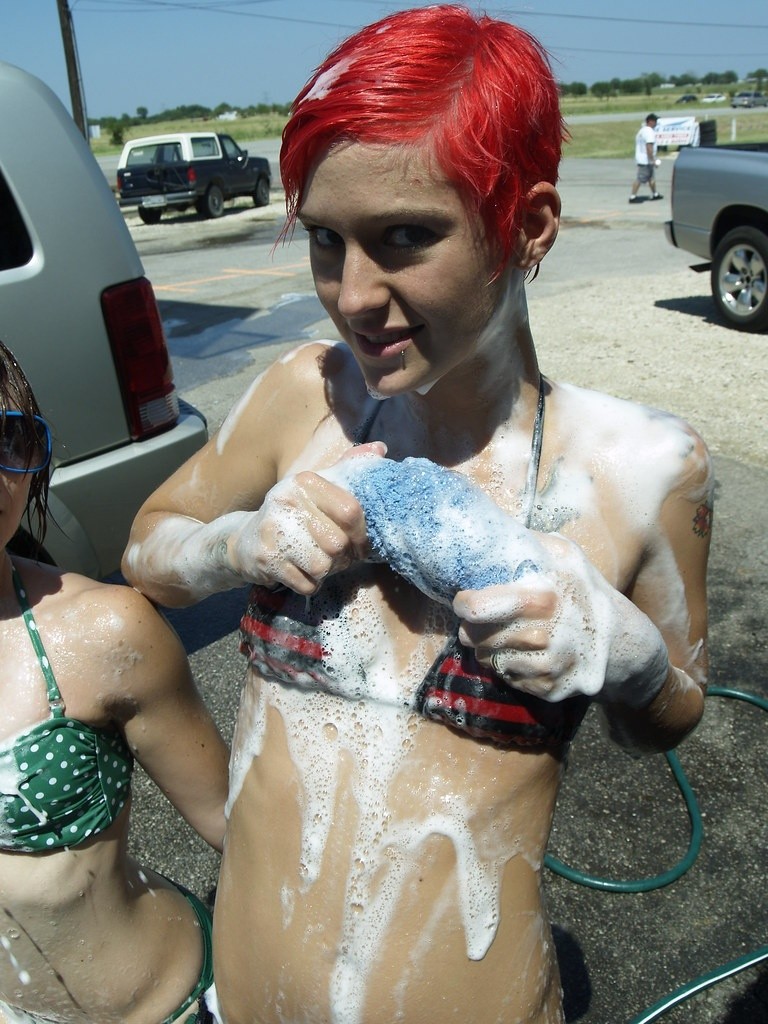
[731,89,768,108]
[700,93,726,105]
[676,95,698,104]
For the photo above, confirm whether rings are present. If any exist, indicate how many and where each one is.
[490,650,508,676]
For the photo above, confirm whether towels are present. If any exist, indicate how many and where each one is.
[344,455,554,606]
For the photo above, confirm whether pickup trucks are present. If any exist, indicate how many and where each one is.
[663,143,768,334]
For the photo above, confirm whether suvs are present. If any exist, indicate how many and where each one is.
[117,132,273,225]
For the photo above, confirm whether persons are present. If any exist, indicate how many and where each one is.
[628,114,665,203]
[123,5,716,1024]
[0,340,230,1024]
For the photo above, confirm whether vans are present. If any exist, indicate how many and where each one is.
[0,64,212,590]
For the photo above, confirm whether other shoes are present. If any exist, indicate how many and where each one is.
[629,195,643,203]
[652,192,664,200]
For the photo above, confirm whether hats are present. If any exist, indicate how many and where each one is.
[645,113,660,122]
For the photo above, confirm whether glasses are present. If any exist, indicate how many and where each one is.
[1,406,54,475]
